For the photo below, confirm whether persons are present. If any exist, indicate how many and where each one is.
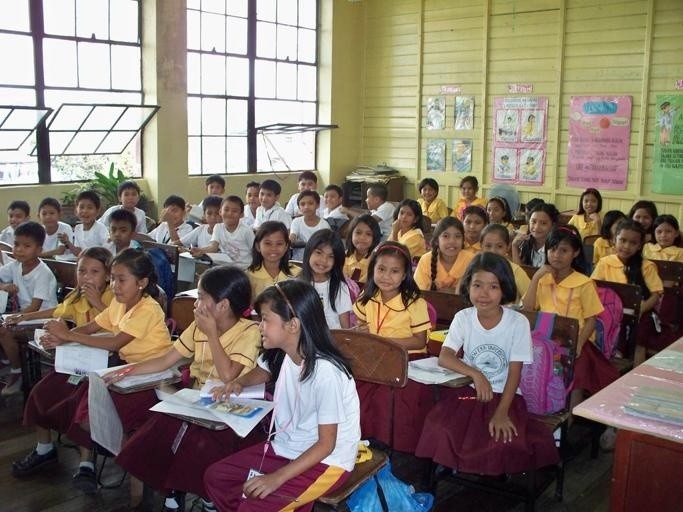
[3,171,681,511]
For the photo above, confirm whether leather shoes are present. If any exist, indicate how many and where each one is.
[72,467,98,495]
[162,488,186,511]
[12,447,56,474]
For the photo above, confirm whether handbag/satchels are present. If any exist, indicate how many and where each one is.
[145,245,174,302]
[594,288,624,359]
[520,312,576,417]
[346,463,434,512]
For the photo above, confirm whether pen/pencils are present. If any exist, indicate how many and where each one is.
[350,322,371,330]
[191,243,192,248]
[45,317,60,337]
[0,315,23,325]
[459,397,477,400]
[119,367,134,376]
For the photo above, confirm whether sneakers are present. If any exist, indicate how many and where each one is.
[0,359,24,395]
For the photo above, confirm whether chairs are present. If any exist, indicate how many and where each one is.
[0,208,682,512]
